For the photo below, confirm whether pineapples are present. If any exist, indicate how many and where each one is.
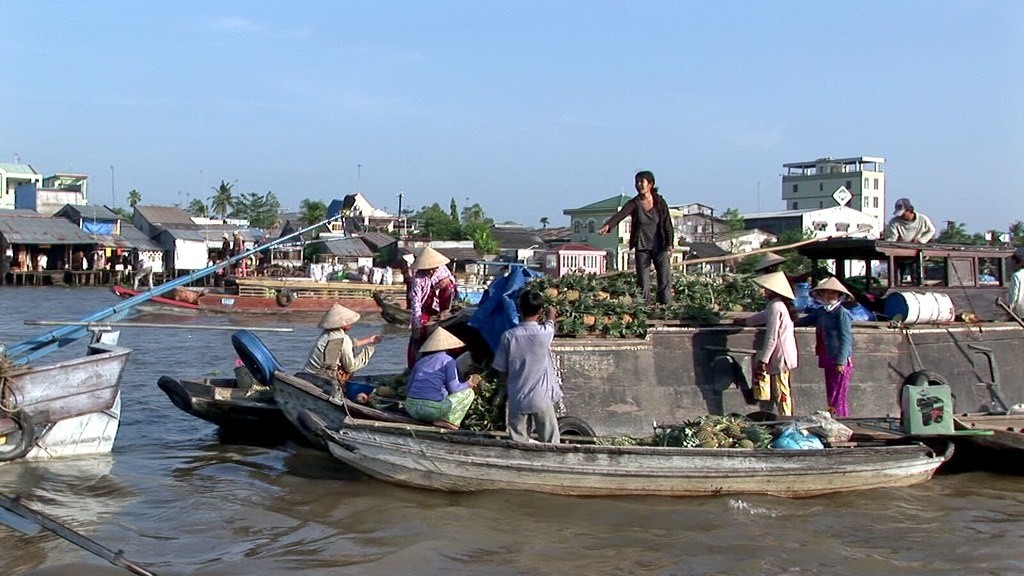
[535,287,635,325]
[674,274,767,312]
[684,414,770,450]
[376,373,482,399]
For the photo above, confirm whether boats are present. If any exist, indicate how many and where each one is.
[951,411,1024,466]
[0,323,135,467]
[229,329,998,497]
[110,234,1024,458]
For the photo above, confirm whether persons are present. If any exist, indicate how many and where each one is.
[405,246,565,445]
[734,271,799,417]
[301,300,382,385]
[882,198,935,286]
[1008,251,1024,324]
[795,276,855,418]
[596,170,674,306]
[208,232,244,286]
[8,246,155,290]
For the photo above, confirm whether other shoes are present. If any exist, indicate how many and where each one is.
[435,420,459,430]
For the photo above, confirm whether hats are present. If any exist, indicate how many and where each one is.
[809,277,855,302]
[411,247,450,269]
[893,198,910,215]
[221,232,230,241]
[318,303,360,329]
[419,326,465,352]
[752,272,796,300]
